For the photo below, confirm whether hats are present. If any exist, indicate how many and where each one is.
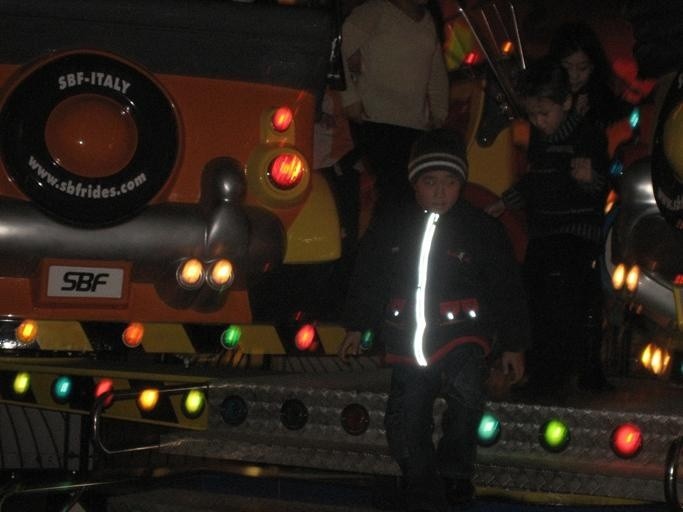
[407,129,467,183]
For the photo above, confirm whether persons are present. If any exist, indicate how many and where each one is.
[329,0,450,223]
[337,129,533,512]
[544,22,634,146]
[313,48,361,323]
[481,57,616,393]
